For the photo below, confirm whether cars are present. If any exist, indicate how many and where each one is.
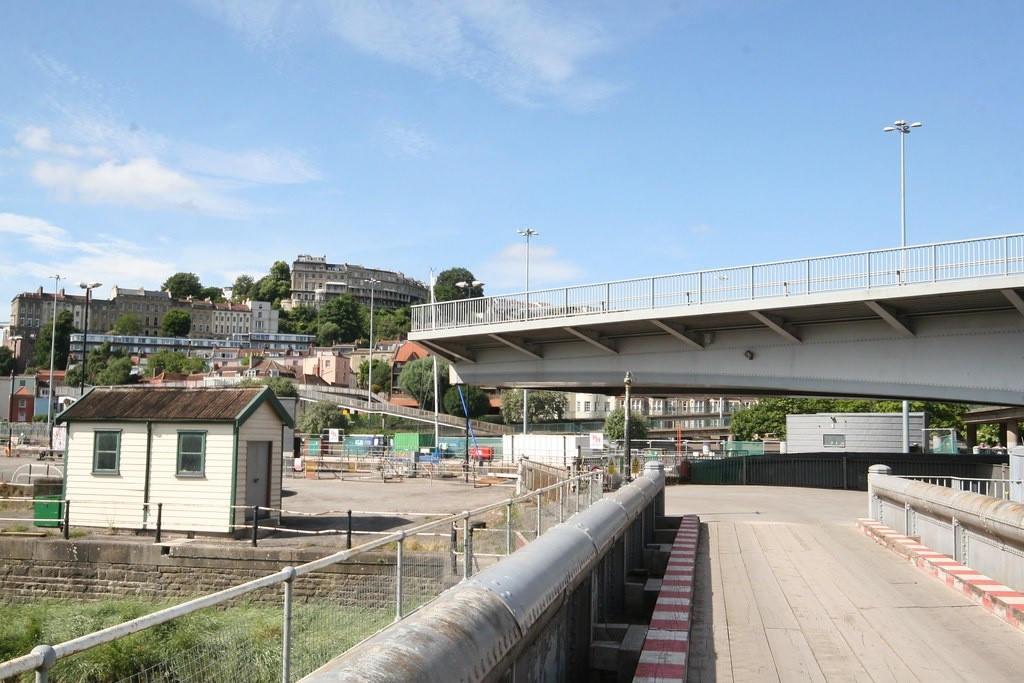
[468,299,602,323]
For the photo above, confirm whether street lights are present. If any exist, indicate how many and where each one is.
[683,440,689,456]
[47,275,66,451]
[456,281,485,322]
[74,281,103,398]
[882,118,922,452]
[623,368,636,483]
[517,228,540,321]
[8,336,23,456]
[365,278,383,428]
[647,441,652,448]
[720,440,725,456]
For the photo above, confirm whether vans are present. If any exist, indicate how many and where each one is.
[641,448,668,462]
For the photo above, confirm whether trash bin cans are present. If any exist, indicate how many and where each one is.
[33,476,64,527]
[679,461,692,481]
